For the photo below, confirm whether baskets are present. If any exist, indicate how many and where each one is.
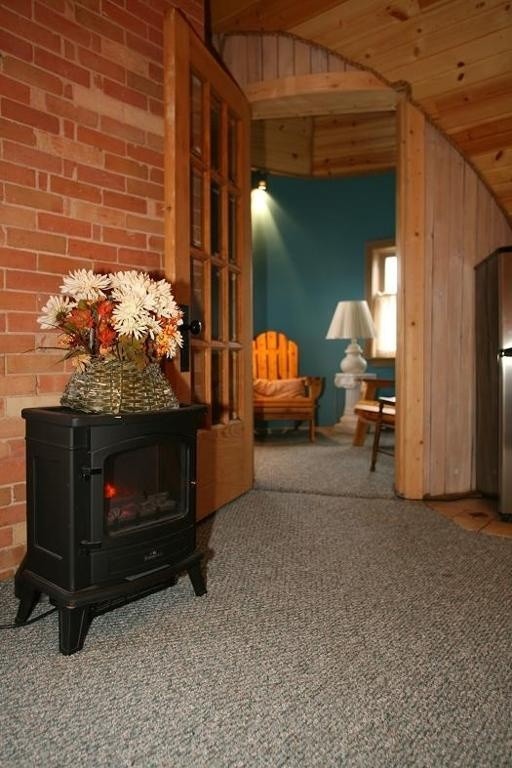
[60,360,178,416]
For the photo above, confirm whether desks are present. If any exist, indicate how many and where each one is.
[333,371,382,437]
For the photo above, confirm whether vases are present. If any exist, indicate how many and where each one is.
[61,345,181,418]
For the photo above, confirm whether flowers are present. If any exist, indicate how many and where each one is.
[25,267,188,364]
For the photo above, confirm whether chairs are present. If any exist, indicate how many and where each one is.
[251,329,324,442]
[347,376,395,447]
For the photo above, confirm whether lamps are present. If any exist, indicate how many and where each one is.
[326,298,378,375]
